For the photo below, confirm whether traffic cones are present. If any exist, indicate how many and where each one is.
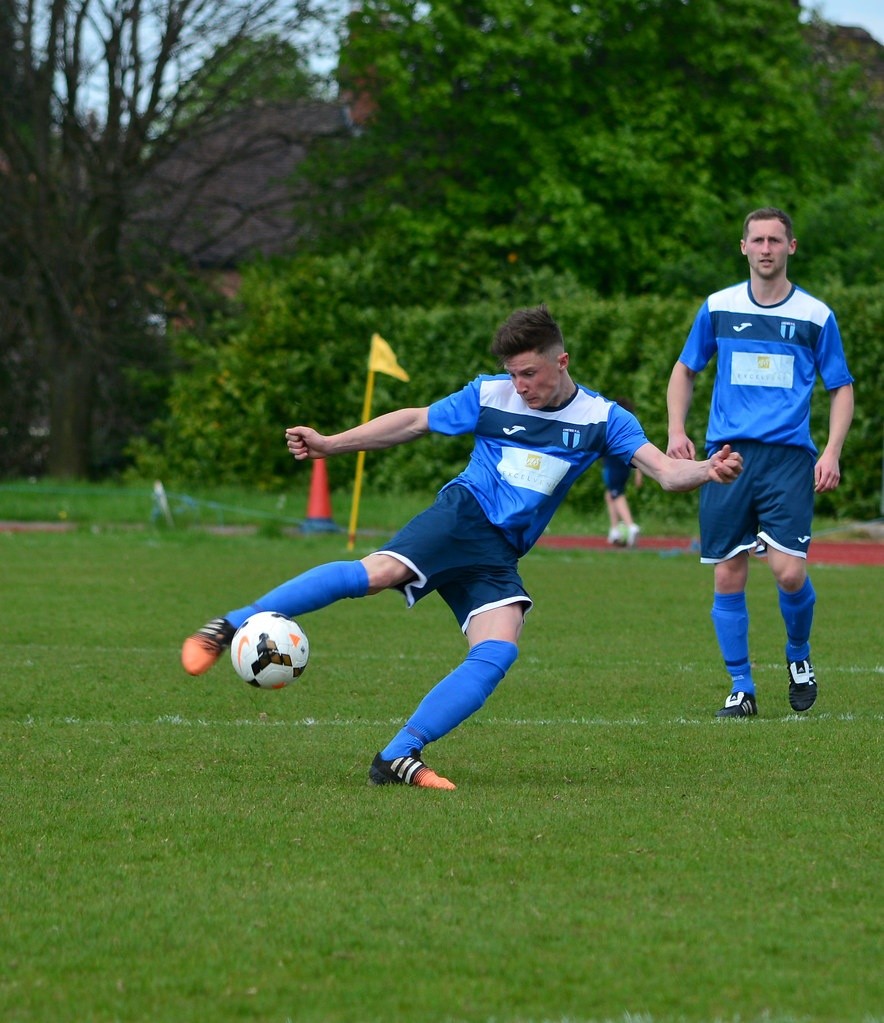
[300,458,341,533]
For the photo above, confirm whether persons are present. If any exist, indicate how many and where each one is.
[181,306,743,791]
[602,456,641,548]
[667,208,854,717]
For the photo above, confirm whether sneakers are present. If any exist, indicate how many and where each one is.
[367,751,456,791]
[786,652,817,711]
[715,691,758,718]
[180,617,237,676]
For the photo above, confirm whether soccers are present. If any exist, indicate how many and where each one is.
[615,521,628,545]
[230,610,311,689]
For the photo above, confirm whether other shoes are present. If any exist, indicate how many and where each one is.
[628,524,639,545]
[608,529,620,543]
